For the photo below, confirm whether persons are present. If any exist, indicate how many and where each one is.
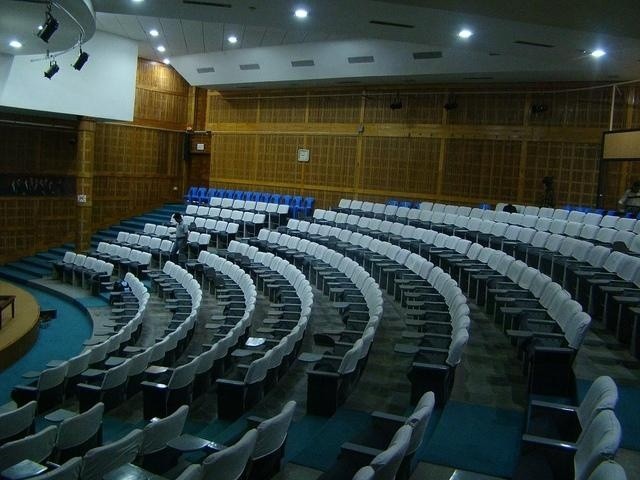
[169,212,189,269]
[616,180,639,213]
[543,176,556,209]
[503,199,517,213]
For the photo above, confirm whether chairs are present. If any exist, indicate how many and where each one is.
[0,185,640,480]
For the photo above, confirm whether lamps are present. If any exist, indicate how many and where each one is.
[29,0,88,80]
